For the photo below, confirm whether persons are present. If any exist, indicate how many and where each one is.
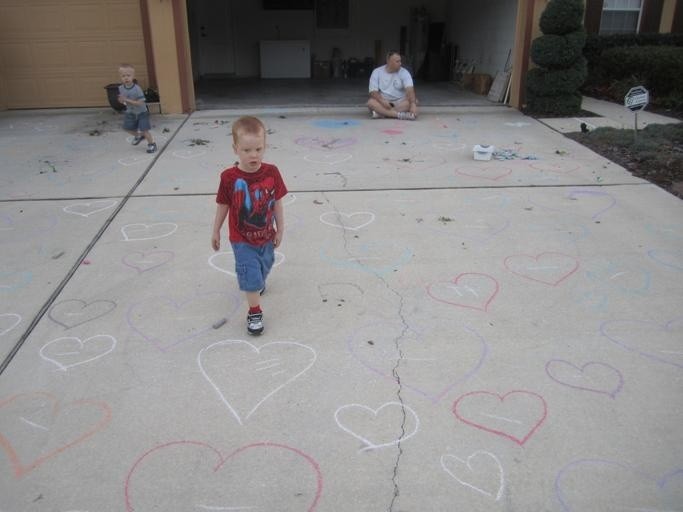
[209,114,289,337]
[367,47,420,120]
[114,61,158,154]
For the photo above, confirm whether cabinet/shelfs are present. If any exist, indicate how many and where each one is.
[260,39,312,79]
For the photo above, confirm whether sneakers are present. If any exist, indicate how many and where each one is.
[147,143,157,153]
[132,136,145,145]
[371,111,384,119]
[247,311,264,335]
[398,112,416,120]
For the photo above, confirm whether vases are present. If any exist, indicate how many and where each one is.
[105,84,127,112]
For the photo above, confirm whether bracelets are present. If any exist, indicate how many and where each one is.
[410,98,417,105]
